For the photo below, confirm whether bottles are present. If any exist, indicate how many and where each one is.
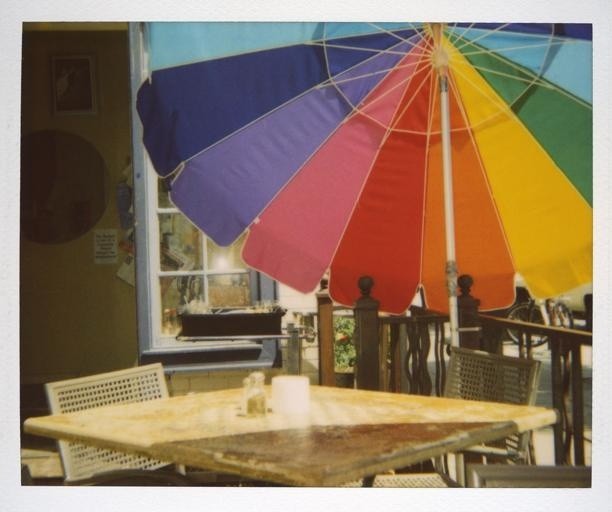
[240,371,268,417]
[161,308,179,336]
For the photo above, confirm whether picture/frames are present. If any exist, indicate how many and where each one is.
[50,54,98,117]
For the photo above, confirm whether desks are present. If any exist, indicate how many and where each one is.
[23,384,558,487]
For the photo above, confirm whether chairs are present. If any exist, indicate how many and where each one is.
[373,345,541,488]
[43,363,250,486]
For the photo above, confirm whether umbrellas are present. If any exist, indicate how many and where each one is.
[135,22,593,488]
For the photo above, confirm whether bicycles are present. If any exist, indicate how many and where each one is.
[505,295,573,346]
